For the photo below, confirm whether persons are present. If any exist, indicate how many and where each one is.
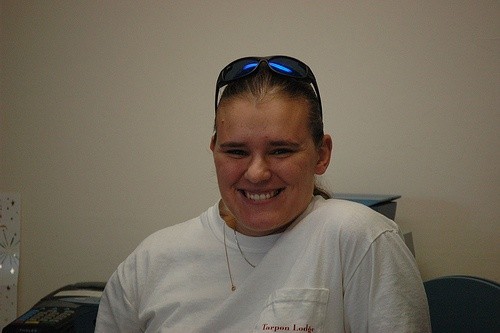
[94,55,431,333]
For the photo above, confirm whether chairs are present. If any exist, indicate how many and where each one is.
[422,275,500,333]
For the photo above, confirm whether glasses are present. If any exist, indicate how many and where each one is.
[215,55,322,122]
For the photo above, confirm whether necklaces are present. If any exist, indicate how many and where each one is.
[223,217,285,291]
